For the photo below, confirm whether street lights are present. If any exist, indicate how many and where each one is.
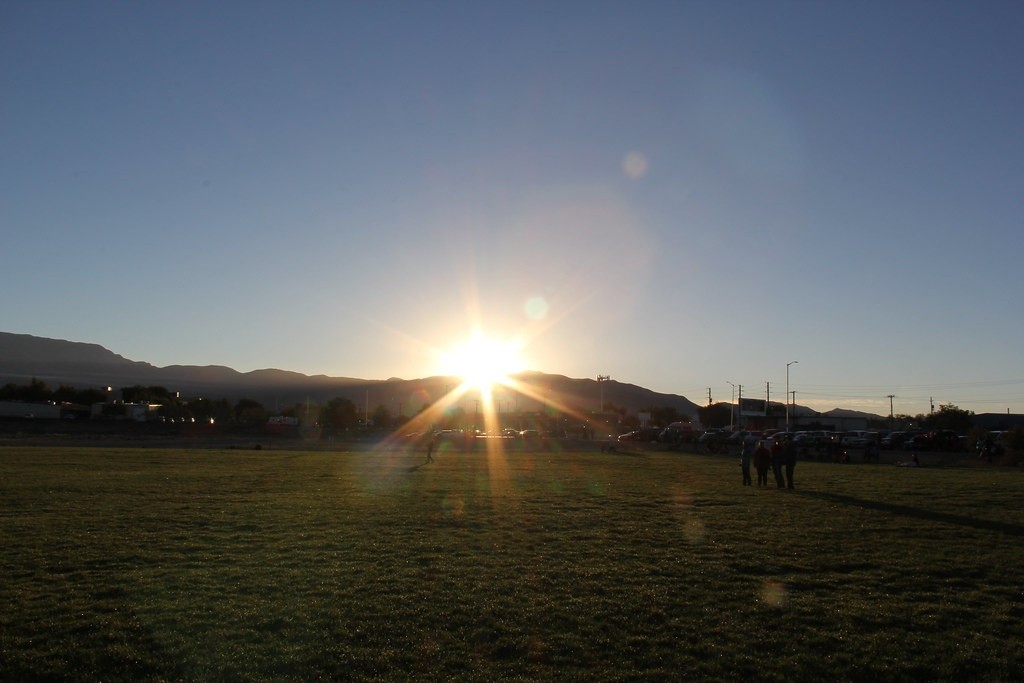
[726,380,735,433]
[785,361,798,431]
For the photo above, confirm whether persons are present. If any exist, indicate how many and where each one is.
[426,438,435,464]
[828,437,1001,468]
[559,426,617,454]
[672,434,798,490]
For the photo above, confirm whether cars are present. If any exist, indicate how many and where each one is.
[406,428,569,439]
[616,420,1024,454]
